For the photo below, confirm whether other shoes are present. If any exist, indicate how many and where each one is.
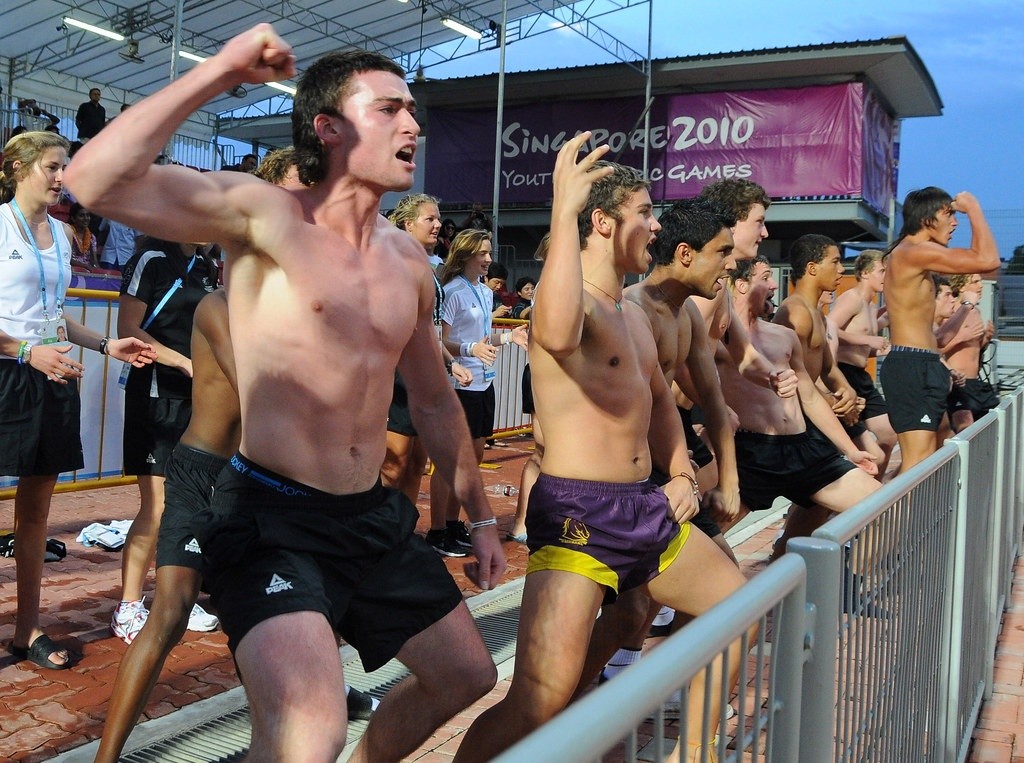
[648,700,733,724]
[647,619,674,638]
[599,666,609,686]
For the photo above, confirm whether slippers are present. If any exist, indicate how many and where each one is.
[505,528,528,544]
[7,634,71,670]
[344,687,374,722]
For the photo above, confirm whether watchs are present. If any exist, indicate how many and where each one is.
[22,343,32,365]
[962,301,975,310]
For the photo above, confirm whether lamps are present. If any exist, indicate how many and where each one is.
[62,15,124,41]
[178,47,207,63]
[439,17,482,40]
[262,80,298,94]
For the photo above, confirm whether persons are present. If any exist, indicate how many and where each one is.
[0,23,1001,763]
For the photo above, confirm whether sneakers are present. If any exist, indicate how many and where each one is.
[184,602,219,632]
[427,527,468,557]
[449,519,473,548]
[110,594,151,646]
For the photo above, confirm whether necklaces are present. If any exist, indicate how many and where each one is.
[649,272,682,309]
[584,279,624,311]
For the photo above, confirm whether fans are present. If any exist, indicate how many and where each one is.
[117,36,144,66]
[224,83,247,98]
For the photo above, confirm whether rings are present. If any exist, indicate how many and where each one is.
[524,341,527,344]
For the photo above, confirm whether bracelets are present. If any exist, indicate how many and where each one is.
[459,342,476,358]
[671,472,699,495]
[447,359,457,375]
[18,340,28,364]
[504,332,509,345]
[469,516,497,528]
[100,336,111,356]
